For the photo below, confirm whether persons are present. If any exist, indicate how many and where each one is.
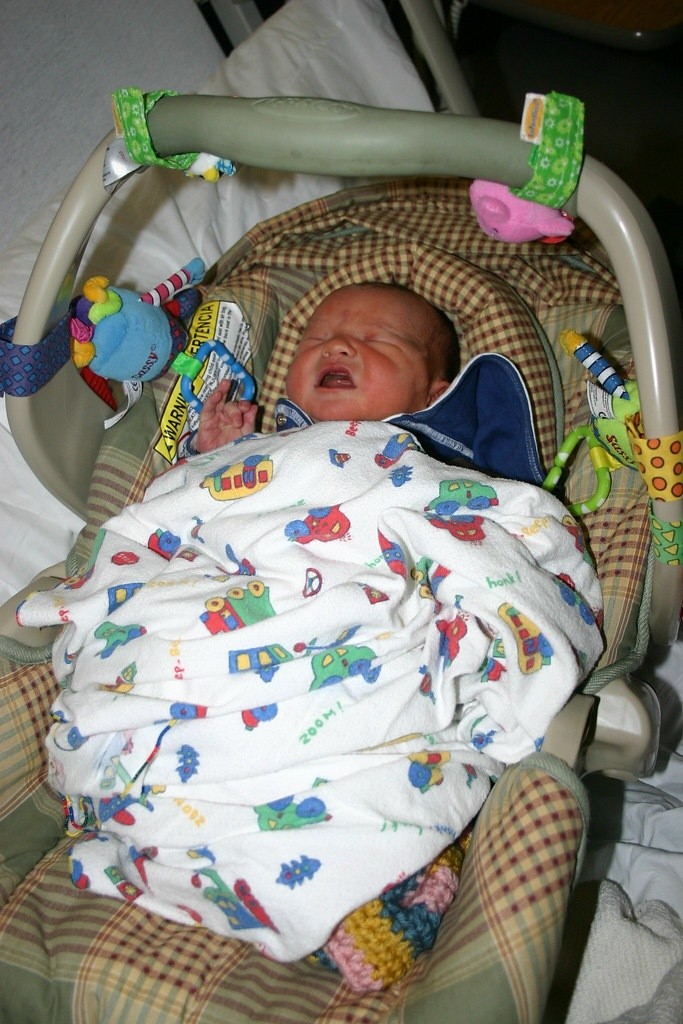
[183,280,462,865]
